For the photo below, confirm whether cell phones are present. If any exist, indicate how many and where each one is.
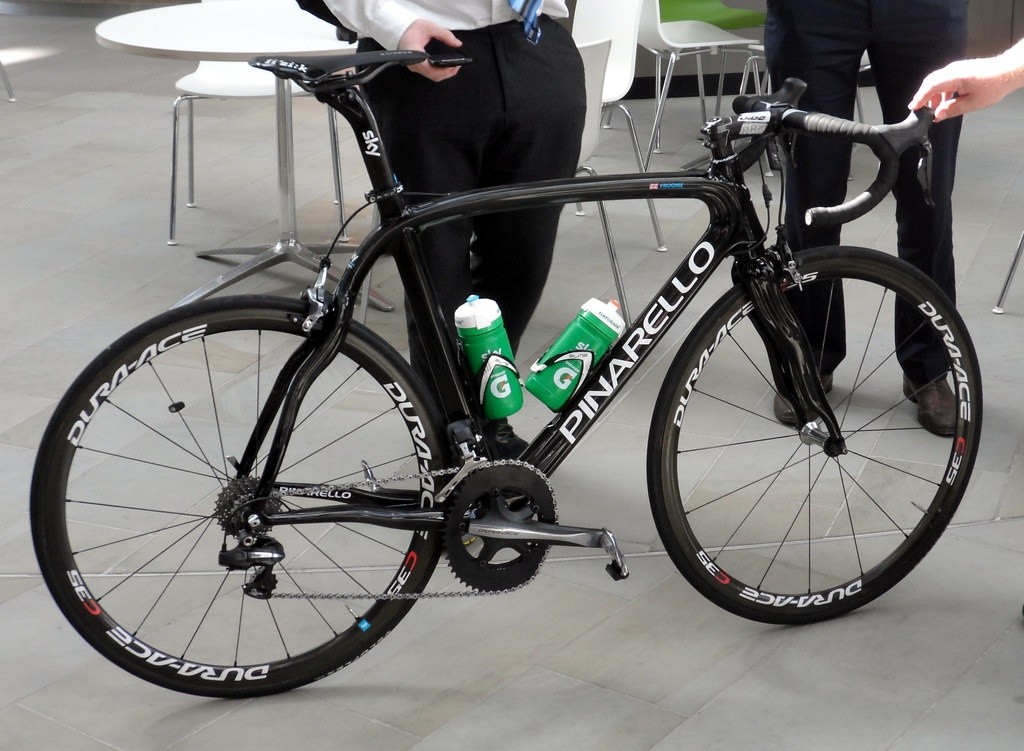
[426,50,473,67]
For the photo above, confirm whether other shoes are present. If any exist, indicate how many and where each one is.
[489,416,530,461]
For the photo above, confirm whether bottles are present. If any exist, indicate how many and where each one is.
[524,298,627,412]
[453,293,522,422]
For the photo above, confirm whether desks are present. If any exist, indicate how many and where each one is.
[582,37,631,331]
[94,0,397,312]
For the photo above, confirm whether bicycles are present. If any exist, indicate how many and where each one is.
[31,55,984,699]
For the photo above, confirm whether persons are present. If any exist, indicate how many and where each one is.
[325,0,587,522]
[764,0,972,437]
[907,35,1023,124]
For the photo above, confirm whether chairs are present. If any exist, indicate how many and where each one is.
[739,45,871,174]
[571,0,670,254]
[603,0,762,152]
[167,0,350,245]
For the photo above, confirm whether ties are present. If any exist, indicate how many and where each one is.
[508,0,544,46]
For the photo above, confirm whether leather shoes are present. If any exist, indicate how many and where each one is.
[902,372,956,435]
[774,371,834,424]
